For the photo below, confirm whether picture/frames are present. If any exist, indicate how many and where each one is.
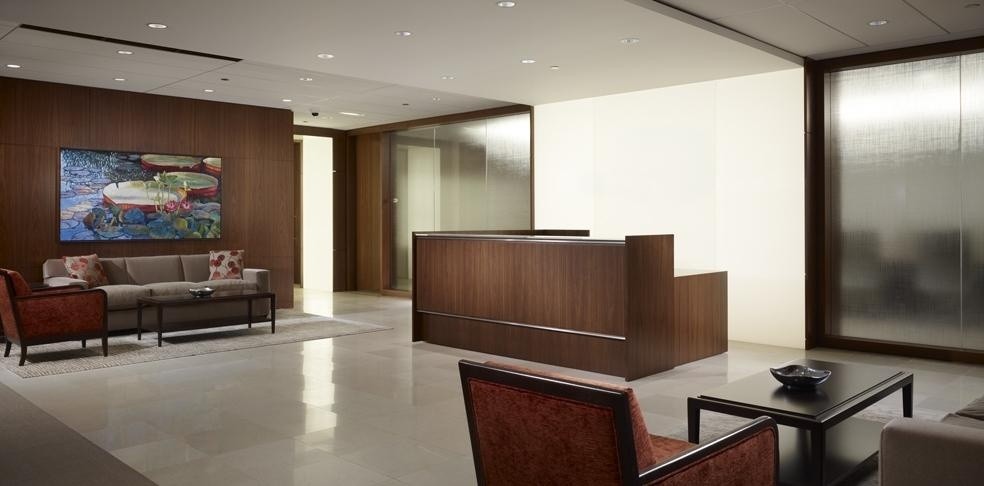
[55,146,224,244]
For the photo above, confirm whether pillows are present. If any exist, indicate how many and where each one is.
[63,252,108,289]
[207,248,245,281]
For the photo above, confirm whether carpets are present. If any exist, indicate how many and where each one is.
[1,308,394,379]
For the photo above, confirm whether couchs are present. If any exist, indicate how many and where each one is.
[43,251,278,351]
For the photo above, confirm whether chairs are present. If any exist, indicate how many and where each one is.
[0,268,110,366]
[878,394,983,485]
[458,357,780,483]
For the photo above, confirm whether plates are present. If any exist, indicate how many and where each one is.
[769,364,832,390]
[187,285,216,296]
